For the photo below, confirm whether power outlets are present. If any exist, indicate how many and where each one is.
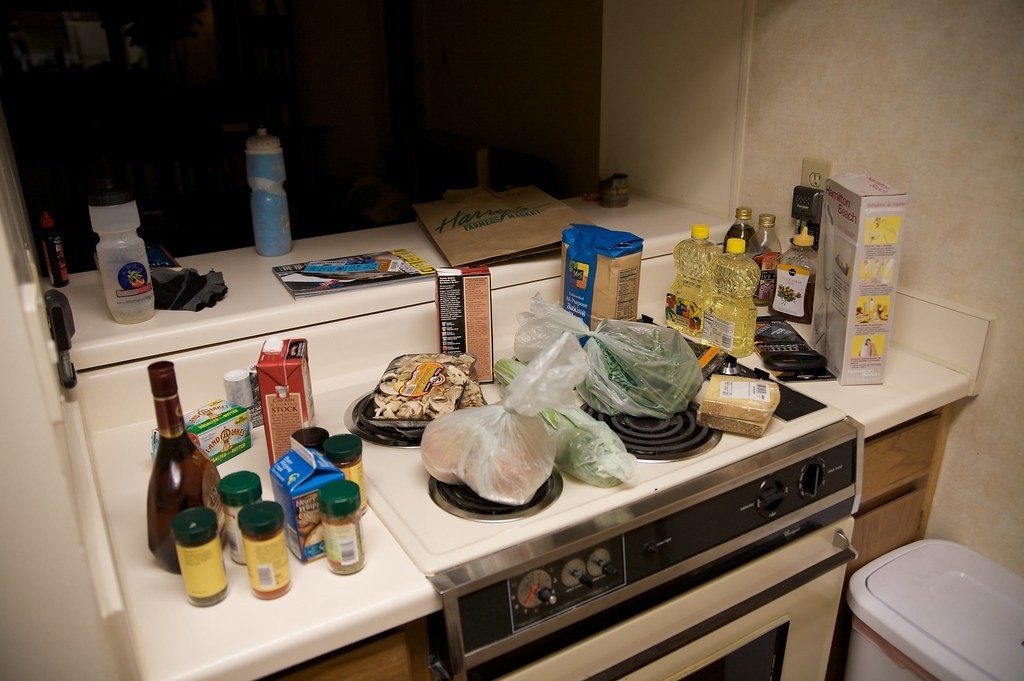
[800,157,831,191]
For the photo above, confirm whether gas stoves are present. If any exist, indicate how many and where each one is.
[307,312,866,669]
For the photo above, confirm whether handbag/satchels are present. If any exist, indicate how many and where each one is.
[412,184,594,270]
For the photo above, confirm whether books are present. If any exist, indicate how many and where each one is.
[272,249,436,300]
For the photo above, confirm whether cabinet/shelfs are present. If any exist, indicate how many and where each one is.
[822,402,954,681]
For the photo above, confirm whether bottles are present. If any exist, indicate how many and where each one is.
[173,427,366,607]
[39,211,70,288]
[664,225,720,337]
[722,206,778,308]
[245,126,293,254]
[145,360,228,573]
[89,175,156,326]
[767,227,818,323]
[701,236,763,355]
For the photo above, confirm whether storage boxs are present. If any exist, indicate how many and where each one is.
[811,172,909,386]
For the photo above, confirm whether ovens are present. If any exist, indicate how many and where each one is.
[490,515,858,681]
